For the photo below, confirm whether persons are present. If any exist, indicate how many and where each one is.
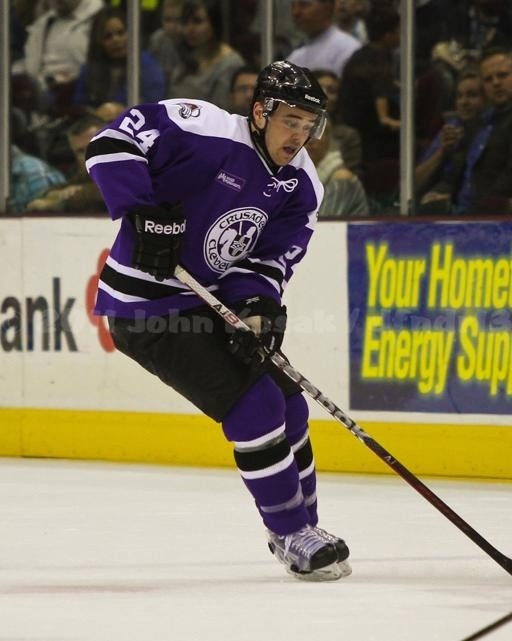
[85,60,348,574]
[1,1,510,221]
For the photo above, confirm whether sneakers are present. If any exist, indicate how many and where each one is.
[264,522,348,569]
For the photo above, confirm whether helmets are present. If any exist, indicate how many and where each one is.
[250,62,328,142]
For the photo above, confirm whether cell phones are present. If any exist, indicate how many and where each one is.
[443,111,458,128]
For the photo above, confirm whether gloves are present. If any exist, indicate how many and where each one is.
[228,296,286,363]
[131,205,190,283]
